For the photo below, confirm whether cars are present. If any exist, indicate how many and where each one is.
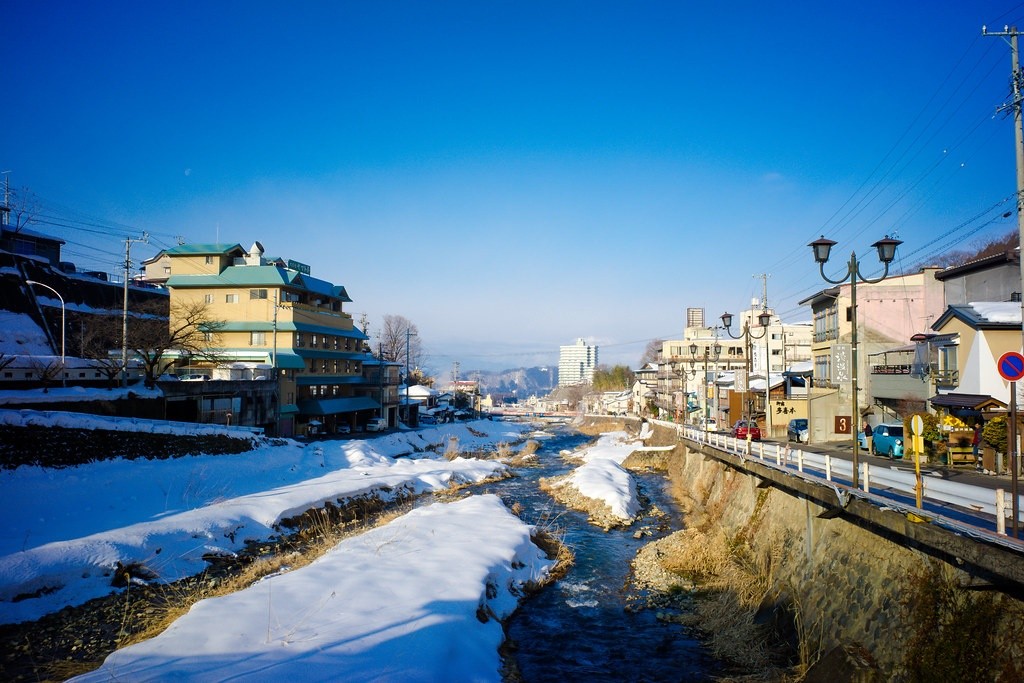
[787,419,808,443]
[858,420,903,448]
[872,423,904,461]
[799,429,809,444]
[699,418,717,433]
[336,421,351,435]
[730,419,760,442]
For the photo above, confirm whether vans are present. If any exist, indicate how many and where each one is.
[366,418,386,433]
[420,416,438,425]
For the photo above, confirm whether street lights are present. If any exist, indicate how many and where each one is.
[25,279,66,388]
[670,359,697,428]
[719,312,772,454]
[690,343,723,442]
[809,234,904,487]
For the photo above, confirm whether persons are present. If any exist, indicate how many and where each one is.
[972,421,983,465]
[862,421,873,455]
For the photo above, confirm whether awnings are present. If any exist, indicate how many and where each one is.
[302,397,381,416]
